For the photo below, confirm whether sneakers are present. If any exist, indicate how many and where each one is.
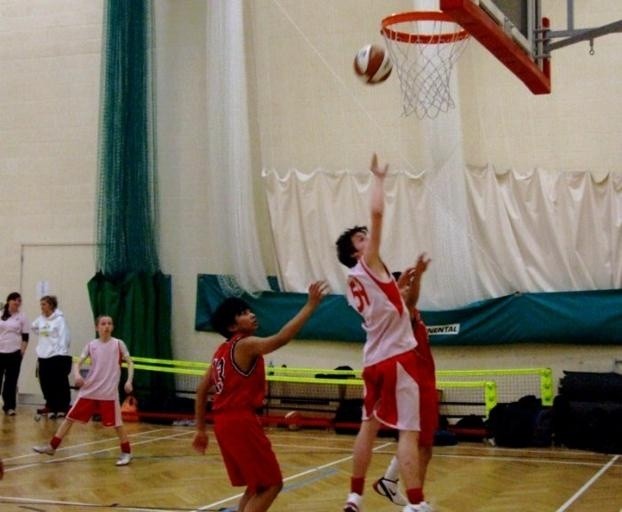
[48,413,56,419]
[343,492,363,512]
[32,446,56,455]
[403,501,436,512]
[37,408,48,414]
[372,477,409,506]
[8,409,16,415]
[56,412,65,417]
[115,452,132,465]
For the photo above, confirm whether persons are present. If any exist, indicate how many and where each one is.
[335,152,439,512]
[29,295,74,418]
[32,313,136,466]
[192,280,333,512]
[370,252,438,507]
[0,292,31,415]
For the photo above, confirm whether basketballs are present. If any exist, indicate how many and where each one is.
[353,45,392,84]
[283,412,302,430]
